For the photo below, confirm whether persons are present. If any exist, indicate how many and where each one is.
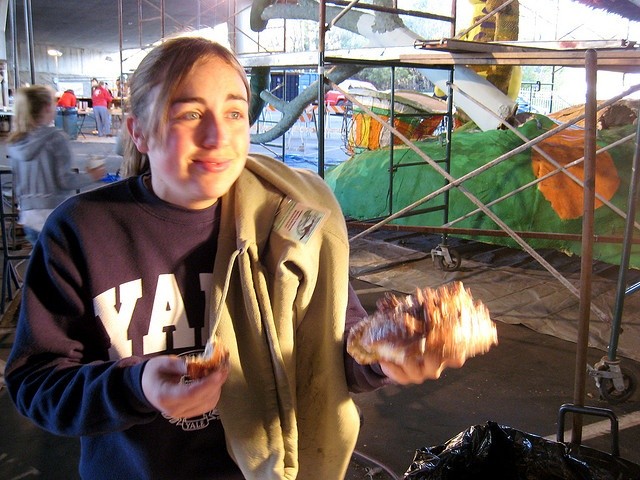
[91,78,98,135]
[57,88,77,115]
[5,85,107,249]
[3,37,466,480]
[92,80,112,137]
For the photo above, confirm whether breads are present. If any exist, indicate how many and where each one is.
[184,337,231,379]
[347,280,498,364]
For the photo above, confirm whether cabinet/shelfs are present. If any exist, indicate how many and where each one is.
[79,97,128,137]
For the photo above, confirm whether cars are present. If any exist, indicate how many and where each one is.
[323,80,378,115]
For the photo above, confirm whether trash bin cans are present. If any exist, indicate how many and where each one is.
[400,403,640,480]
